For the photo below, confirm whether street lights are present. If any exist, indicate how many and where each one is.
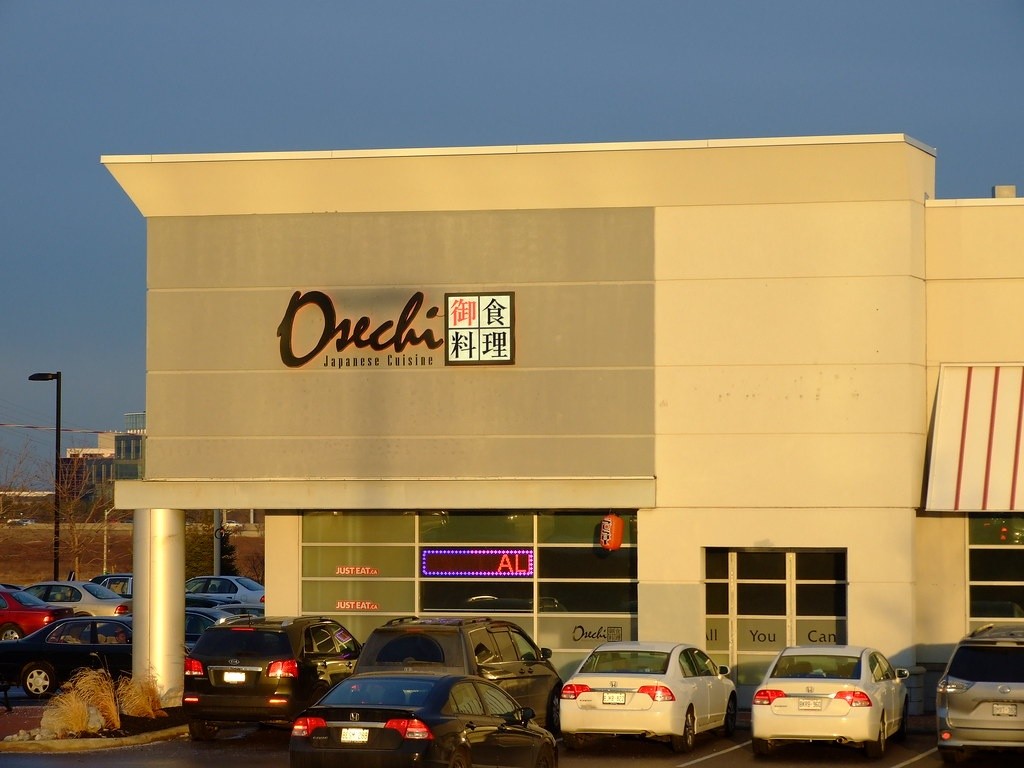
[28,370,63,582]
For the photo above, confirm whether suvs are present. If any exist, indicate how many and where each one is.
[351,613,564,735]
[935,622,1024,764]
[180,613,363,740]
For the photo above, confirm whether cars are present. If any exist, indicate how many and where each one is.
[0,583,74,642]
[288,672,560,768]
[88,574,133,598]
[558,641,738,754]
[185,607,239,651]
[185,575,265,605]
[0,616,133,698]
[14,580,133,617]
[750,644,910,760]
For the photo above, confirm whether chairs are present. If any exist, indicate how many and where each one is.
[791,661,813,677]
